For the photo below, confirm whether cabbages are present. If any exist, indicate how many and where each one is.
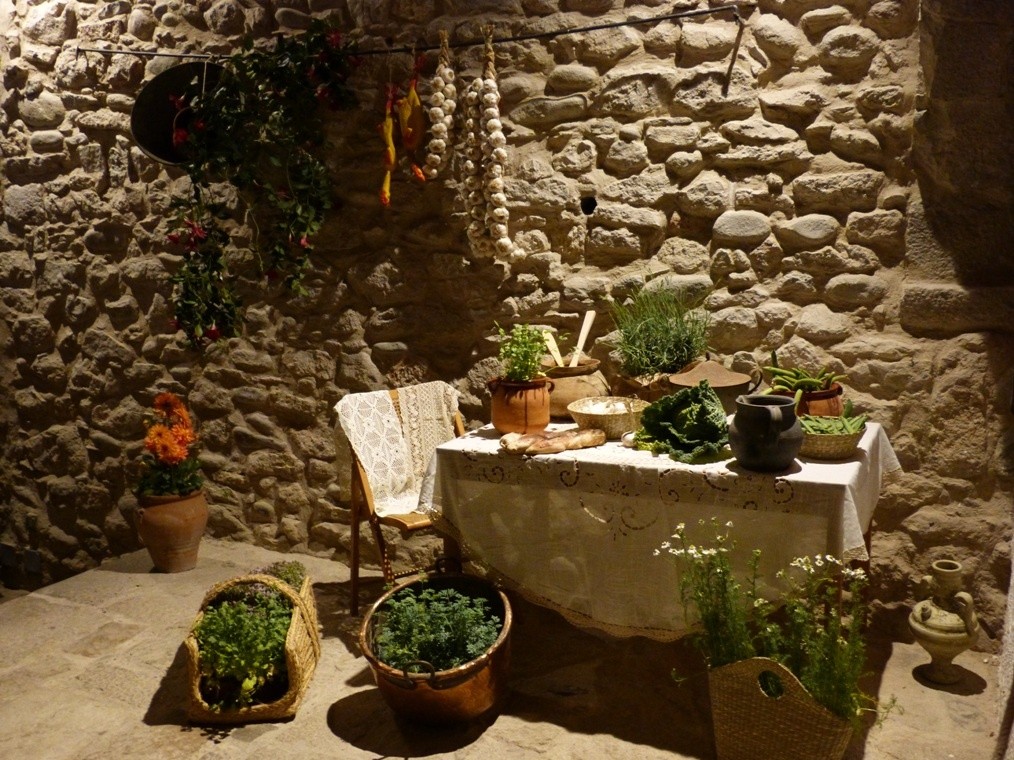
[620,378,731,463]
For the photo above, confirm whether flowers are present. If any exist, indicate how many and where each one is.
[139,393,205,497]
[650,511,893,728]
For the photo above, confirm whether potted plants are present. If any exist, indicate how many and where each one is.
[183,561,316,723]
[483,319,555,434]
[362,568,513,717]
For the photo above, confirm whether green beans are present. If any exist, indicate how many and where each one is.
[797,406,869,434]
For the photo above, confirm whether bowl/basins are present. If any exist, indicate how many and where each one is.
[130,62,239,166]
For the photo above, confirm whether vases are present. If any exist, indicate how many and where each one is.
[136,494,208,574]
